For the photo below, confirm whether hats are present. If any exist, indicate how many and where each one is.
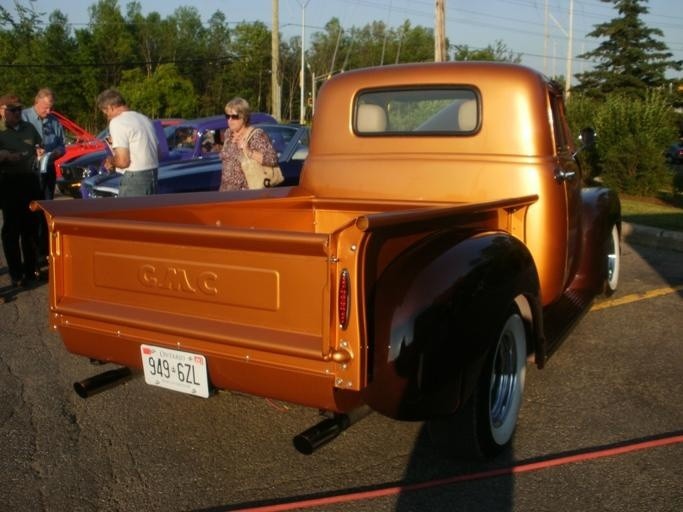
[0,94,25,110]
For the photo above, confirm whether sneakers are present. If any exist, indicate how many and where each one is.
[12,269,45,287]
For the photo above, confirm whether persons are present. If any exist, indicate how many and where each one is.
[175,126,210,153]
[20,87,66,267]
[94,90,160,197]
[220,96,279,190]
[0,94,48,288]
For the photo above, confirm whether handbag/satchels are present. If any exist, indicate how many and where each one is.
[241,149,285,190]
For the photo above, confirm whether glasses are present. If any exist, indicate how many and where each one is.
[225,113,240,120]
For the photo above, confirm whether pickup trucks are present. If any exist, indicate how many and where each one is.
[27,61,624,466]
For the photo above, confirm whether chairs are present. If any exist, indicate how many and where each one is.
[458,99,477,131]
[357,104,387,132]
